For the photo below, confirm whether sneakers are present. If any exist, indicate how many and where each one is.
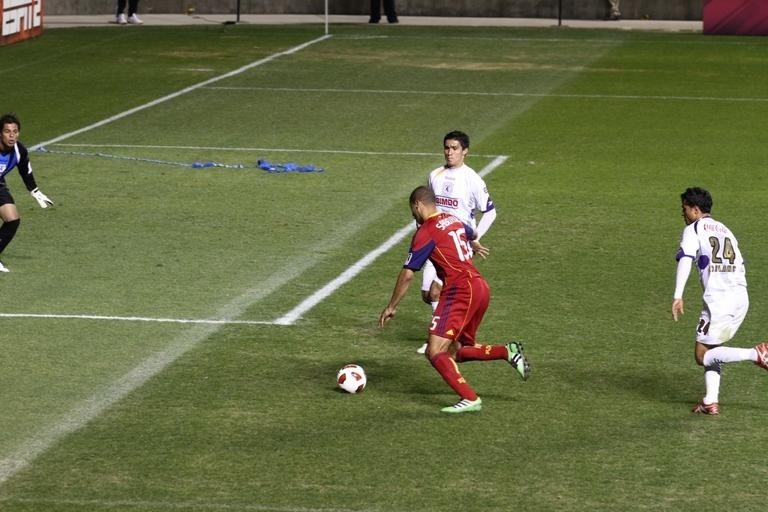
[754,341,768,371]
[416,343,428,354]
[116,13,143,25]
[0,260,10,273]
[690,398,720,416]
[504,340,531,381]
[369,15,399,24]
[439,396,483,415]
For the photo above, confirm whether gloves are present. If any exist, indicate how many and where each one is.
[30,187,55,209]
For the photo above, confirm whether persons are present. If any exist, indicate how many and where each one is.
[116,0,143,25]
[673,185,768,414]
[0,112,55,272]
[367,0,399,23]
[379,184,531,414]
[415,129,497,356]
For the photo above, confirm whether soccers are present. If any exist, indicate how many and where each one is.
[337,364,367,393]
